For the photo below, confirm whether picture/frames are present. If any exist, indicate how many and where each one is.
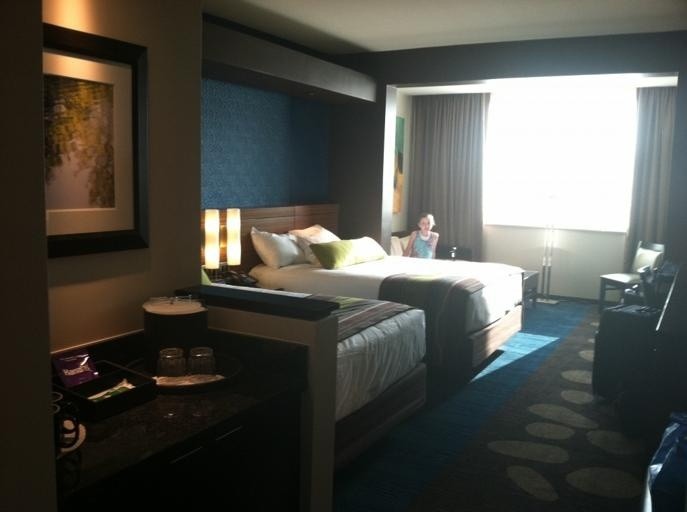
[42,20,150,260]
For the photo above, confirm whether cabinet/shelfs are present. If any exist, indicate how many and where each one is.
[84,395,300,512]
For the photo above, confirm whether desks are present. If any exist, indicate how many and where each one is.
[524,270,540,309]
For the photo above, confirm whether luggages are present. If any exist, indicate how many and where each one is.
[590,302,663,400]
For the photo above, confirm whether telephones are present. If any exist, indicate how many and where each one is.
[224,270,258,286]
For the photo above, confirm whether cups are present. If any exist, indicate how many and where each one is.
[52,392,80,460]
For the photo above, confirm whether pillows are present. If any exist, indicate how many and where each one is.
[250,223,389,269]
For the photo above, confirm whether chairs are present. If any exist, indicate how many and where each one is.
[600,240,667,314]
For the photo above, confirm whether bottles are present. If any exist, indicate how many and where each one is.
[158,345,214,377]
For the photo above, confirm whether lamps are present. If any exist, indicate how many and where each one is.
[204,208,243,282]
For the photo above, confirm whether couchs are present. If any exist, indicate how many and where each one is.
[391,231,472,261]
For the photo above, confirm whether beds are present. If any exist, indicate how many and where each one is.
[294,291,434,468]
[250,255,524,379]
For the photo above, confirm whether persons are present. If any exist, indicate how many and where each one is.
[403,214,439,259]
